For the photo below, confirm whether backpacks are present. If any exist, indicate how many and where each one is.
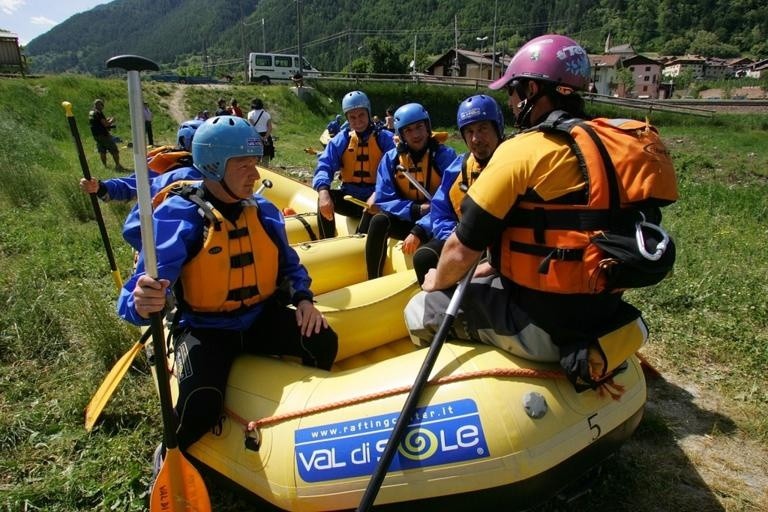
[524,109,680,295]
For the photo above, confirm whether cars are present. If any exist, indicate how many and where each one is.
[681,96,695,100]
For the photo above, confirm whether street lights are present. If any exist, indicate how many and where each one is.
[476,35,488,80]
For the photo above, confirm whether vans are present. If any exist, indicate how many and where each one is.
[249,52,321,85]
[706,96,721,100]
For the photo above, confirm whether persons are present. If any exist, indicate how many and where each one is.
[402,34,623,362]
[312,90,397,241]
[412,94,506,291]
[225,74,232,83]
[366,102,457,280]
[79,97,275,251]
[291,70,303,87]
[117,114,338,489]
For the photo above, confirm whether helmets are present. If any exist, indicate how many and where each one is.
[485,33,593,92]
[191,114,266,182]
[176,119,207,151]
[341,90,372,124]
[455,93,506,141]
[392,102,433,145]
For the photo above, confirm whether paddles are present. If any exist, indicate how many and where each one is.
[106,56,213,512]
[86,296,176,430]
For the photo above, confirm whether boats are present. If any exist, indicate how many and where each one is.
[145,162,650,511]
